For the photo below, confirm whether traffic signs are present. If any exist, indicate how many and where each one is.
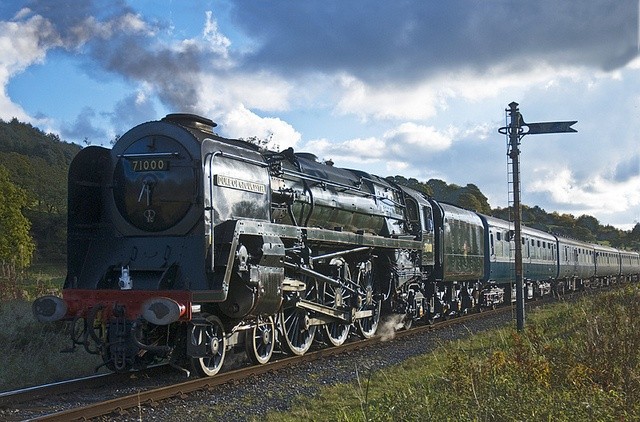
[522,120,578,135]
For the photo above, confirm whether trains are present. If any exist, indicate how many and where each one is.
[34,113,639,376]
